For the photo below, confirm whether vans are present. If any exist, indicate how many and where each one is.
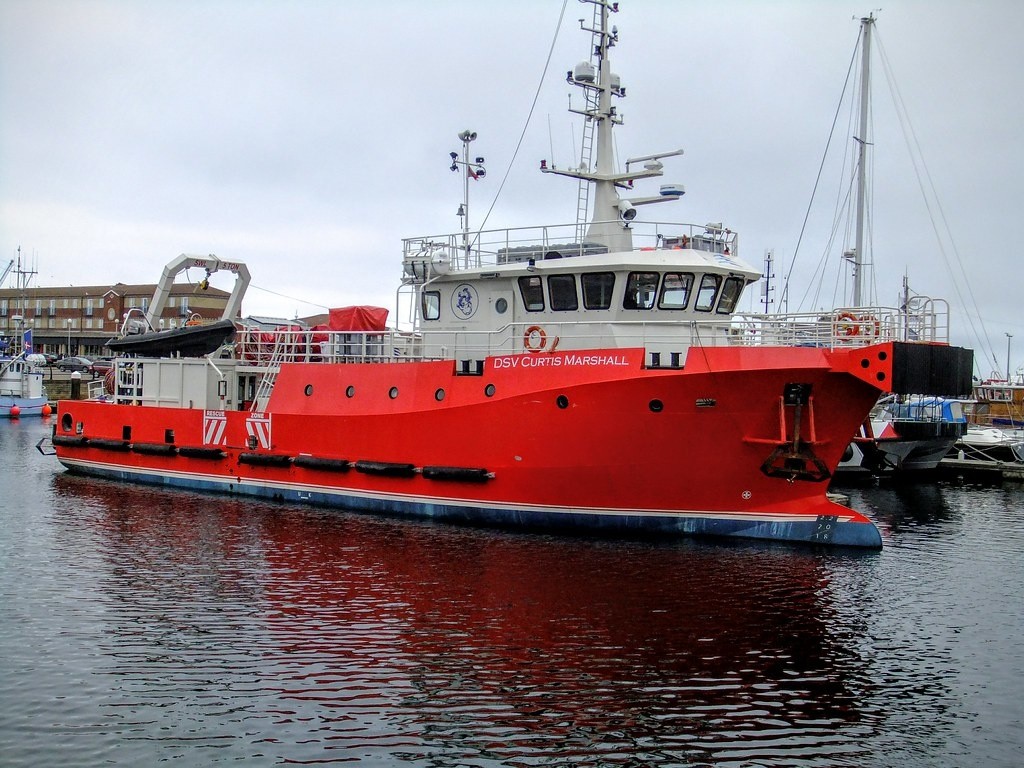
[97,356,123,361]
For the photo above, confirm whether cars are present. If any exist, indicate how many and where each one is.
[54,357,93,373]
[89,361,117,378]
[41,353,60,367]
[76,354,96,361]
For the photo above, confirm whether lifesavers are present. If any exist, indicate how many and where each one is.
[857,315,879,344]
[834,312,859,341]
[524,326,547,353]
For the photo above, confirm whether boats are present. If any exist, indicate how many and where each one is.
[928,427,1024,468]
[0,245,53,414]
[968,332,1024,427]
[36,0,893,558]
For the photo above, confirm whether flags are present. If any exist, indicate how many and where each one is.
[468,166,480,181]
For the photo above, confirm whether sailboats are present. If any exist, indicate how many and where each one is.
[730,11,968,472]
